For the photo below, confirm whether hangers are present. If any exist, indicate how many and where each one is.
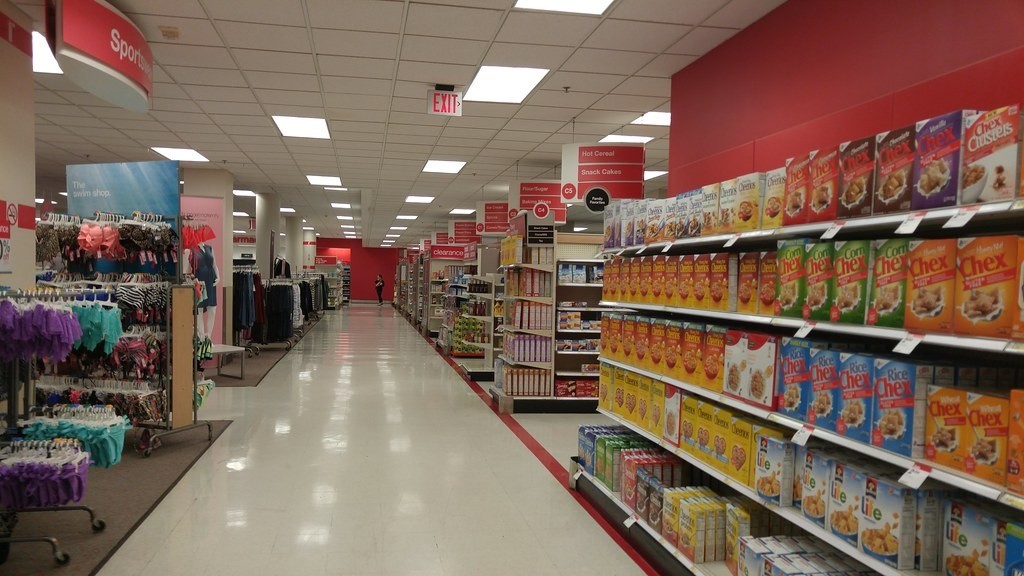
[233,265,321,286]
[0,212,172,472]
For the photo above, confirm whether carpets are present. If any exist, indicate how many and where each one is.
[205,313,324,387]
[0,420,233,576]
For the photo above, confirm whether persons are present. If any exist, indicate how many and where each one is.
[375,274,385,305]
[182,241,220,348]
[275,253,291,279]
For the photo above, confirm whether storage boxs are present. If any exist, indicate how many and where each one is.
[494,102,1023,576]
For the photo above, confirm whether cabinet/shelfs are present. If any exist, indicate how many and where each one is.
[315,210,639,414]
[575,197,1024,576]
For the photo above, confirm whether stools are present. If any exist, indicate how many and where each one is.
[209,344,245,380]
